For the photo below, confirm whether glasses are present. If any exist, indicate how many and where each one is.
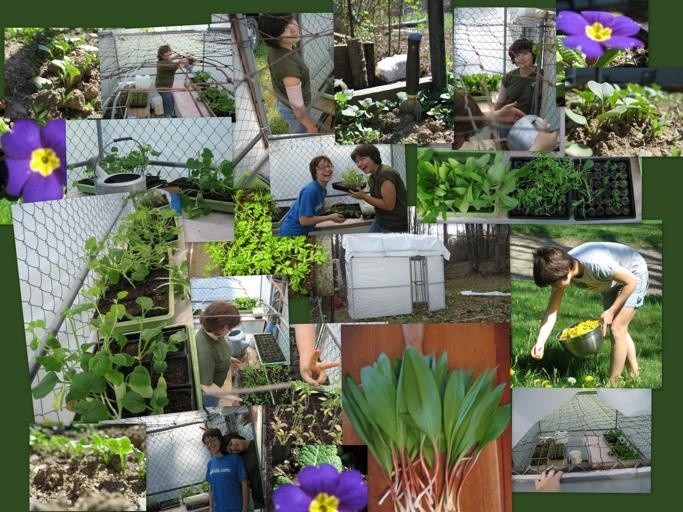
[315,164,335,171]
[204,438,219,446]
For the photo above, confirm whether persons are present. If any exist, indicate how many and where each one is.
[261,12,319,136]
[224,432,264,511]
[196,301,244,405]
[496,41,547,139]
[154,44,185,119]
[453,86,557,153]
[533,468,562,492]
[348,145,405,233]
[202,427,248,511]
[280,155,344,236]
[292,325,340,388]
[531,240,648,388]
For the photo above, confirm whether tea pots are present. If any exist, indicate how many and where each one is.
[94,137,148,195]
[153,96,165,115]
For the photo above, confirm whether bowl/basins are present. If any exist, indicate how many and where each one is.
[557,317,602,356]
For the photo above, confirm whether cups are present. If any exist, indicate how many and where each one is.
[570,451,582,464]
